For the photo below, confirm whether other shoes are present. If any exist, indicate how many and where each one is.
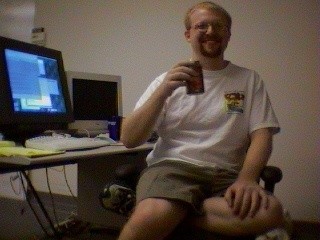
[97,178,137,218]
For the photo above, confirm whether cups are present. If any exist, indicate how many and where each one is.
[108,116,122,141]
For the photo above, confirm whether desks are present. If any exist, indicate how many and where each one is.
[0,140,153,240]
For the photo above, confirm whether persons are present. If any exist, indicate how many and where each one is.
[99,1,283,240]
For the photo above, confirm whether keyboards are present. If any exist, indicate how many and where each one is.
[24,135,110,151]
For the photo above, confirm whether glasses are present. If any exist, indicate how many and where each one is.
[190,24,230,33]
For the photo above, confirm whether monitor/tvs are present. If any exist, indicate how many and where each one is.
[64,70,123,137]
[0,36,75,142]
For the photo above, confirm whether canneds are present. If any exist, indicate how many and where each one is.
[185,60,204,94]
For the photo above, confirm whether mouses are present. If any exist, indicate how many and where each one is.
[95,135,116,144]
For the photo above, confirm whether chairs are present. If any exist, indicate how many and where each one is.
[113,162,283,193]
